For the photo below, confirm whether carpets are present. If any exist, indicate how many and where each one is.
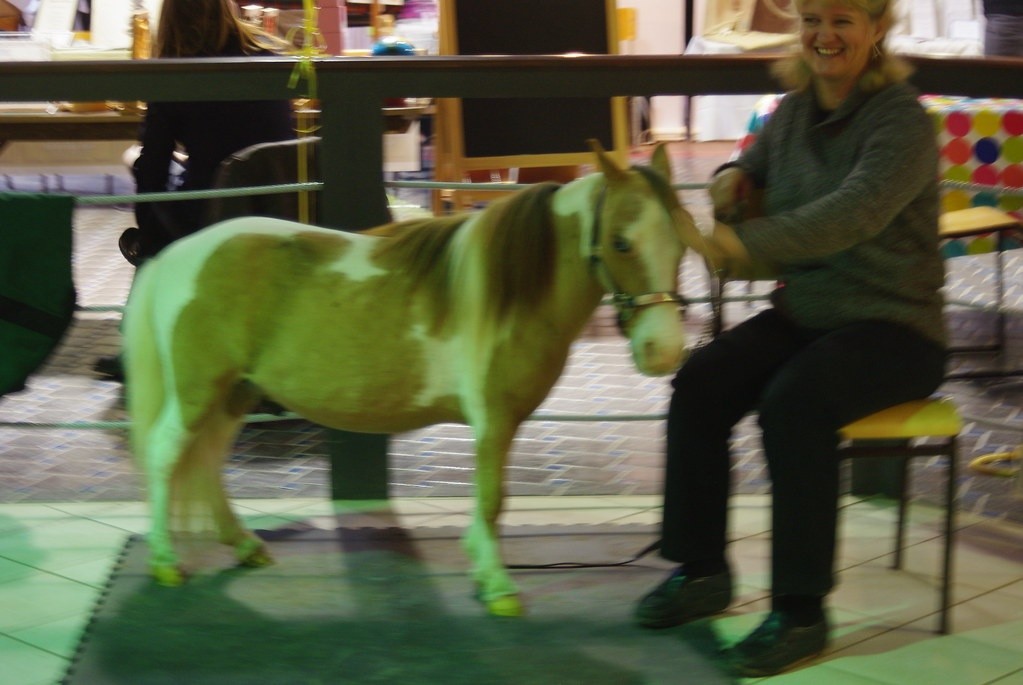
[56,520,742,685]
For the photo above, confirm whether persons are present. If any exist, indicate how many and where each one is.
[90,0,311,380]
[634,0,949,676]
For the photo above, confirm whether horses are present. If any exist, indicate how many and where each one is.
[122,134,693,619]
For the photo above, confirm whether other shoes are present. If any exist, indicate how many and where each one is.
[95,355,124,380]
[634,567,731,628]
[118,227,140,267]
[723,615,825,678]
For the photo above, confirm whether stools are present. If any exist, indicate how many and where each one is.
[938,208,1022,383]
[831,401,963,636]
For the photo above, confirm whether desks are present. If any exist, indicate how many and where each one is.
[731,92,1023,260]
[0,103,421,146]
